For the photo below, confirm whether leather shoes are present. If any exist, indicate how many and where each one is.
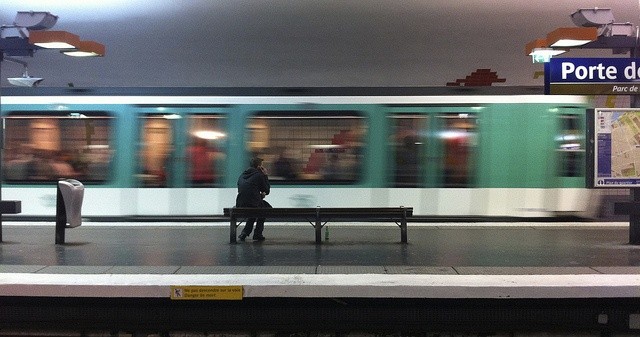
[253,231,265,240]
[238,232,246,240]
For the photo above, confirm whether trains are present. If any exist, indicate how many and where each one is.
[0,87,611,220]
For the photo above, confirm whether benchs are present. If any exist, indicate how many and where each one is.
[2,202,22,239]
[613,201,639,242]
[224,205,414,244]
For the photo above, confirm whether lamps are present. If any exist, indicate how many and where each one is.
[525,38,570,62]
[61,41,104,59]
[548,28,598,50]
[29,30,81,51]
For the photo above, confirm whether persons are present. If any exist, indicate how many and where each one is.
[236,158,270,240]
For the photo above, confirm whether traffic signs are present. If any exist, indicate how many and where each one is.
[550,58,639,96]
